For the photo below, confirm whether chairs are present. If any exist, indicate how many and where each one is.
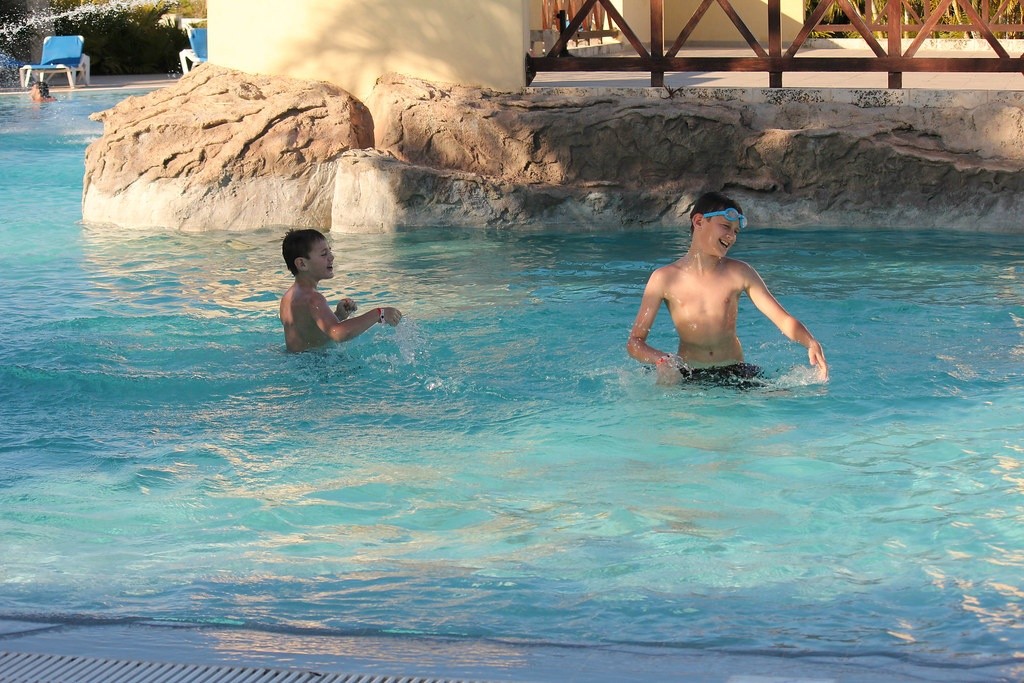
[18,35,90,90]
[179,27,208,75]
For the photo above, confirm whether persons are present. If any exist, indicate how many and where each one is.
[31,81,57,111]
[280,228,403,354]
[626,190,829,395]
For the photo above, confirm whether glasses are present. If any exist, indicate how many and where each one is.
[703,208,748,229]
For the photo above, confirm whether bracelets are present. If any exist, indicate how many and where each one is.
[375,307,386,324]
[654,354,673,366]
